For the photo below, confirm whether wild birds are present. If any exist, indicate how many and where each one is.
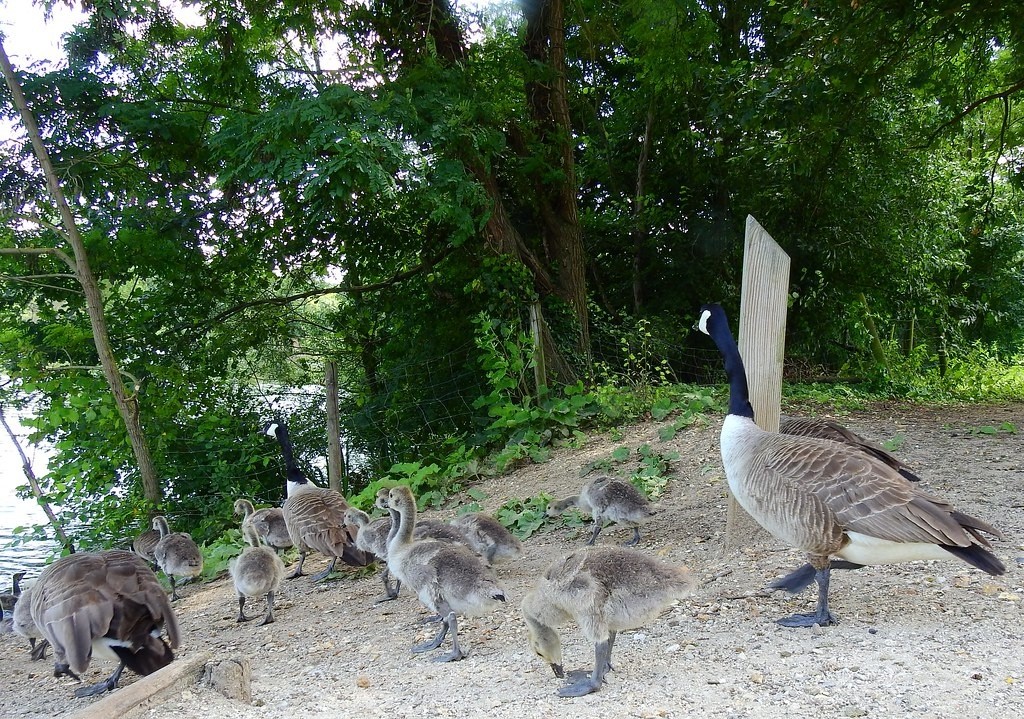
[691,303,1007,627]
[151,516,204,602]
[226,420,524,661]
[0,549,181,699]
[542,475,657,548]
[130,530,193,572]
[520,544,696,699]
